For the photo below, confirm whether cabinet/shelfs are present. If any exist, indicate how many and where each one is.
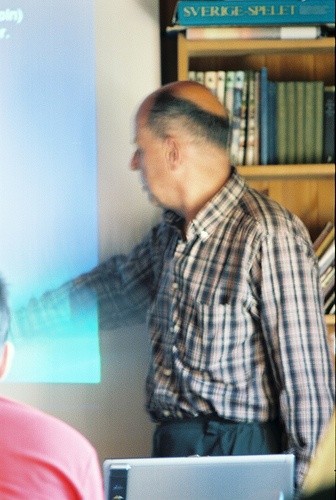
[158,1,336,384]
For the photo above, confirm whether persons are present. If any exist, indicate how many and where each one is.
[15,79,336,499]
[0,279,106,500]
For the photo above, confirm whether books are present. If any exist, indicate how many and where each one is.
[188,68,336,166]
[308,218,336,318]
[187,25,321,40]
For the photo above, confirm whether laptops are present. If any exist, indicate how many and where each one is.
[103,453,295,500]
